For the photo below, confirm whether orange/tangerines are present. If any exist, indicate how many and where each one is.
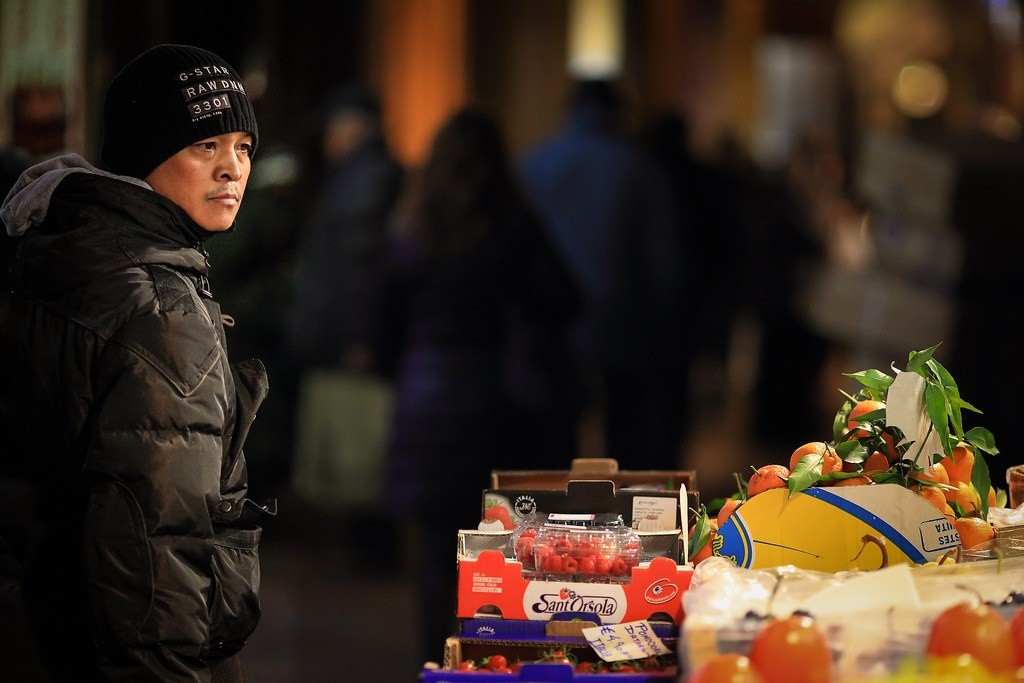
[686,400,996,567]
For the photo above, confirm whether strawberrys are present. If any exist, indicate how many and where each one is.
[485,505,514,530]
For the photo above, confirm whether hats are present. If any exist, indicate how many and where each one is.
[100,44,259,181]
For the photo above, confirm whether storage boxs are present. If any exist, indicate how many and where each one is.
[455,530,695,626]
[461,609,678,637]
[481,459,701,531]
[421,635,680,683]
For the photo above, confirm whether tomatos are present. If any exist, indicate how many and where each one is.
[690,600,1024,683]
[456,649,677,675]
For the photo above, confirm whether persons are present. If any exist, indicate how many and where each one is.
[0,34,273,683]
[194,3,1024,597]
